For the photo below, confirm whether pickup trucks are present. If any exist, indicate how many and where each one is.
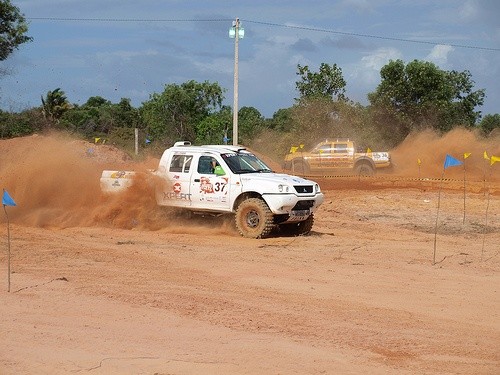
[284,138,391,183]
[99,141,325,239]
